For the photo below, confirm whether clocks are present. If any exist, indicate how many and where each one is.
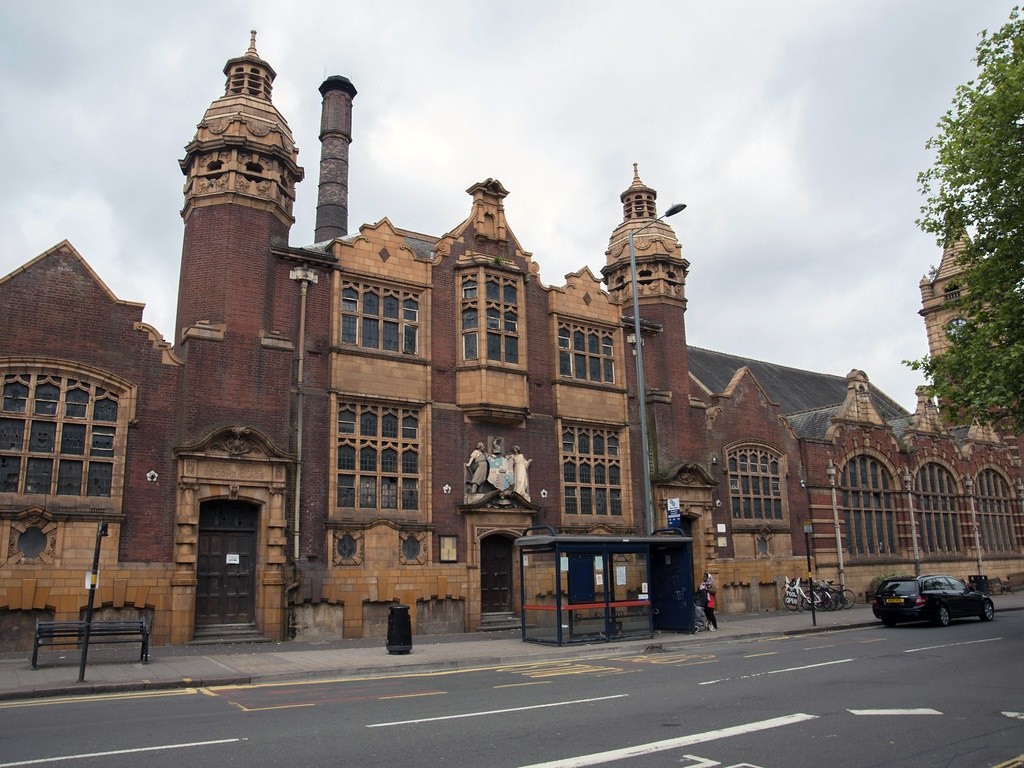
[943,314,969,341]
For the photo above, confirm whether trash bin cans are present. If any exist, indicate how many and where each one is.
[386,605,413,654]
[969,575,989,592]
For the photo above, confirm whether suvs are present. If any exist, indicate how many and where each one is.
[872,573,995,628]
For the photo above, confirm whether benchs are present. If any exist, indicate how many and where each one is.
[30,616,151,670]
[987,576,1014,596]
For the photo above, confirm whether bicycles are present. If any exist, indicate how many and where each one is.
[782,579,856,613]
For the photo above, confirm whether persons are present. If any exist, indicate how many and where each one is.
[466,441,490,492]
[697,572,718,632]
[505,445,533,503]
[493,438,501,451]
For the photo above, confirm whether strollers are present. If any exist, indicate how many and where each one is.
[693,591,710,633]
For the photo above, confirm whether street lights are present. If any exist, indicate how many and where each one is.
[628,202,690,534]
